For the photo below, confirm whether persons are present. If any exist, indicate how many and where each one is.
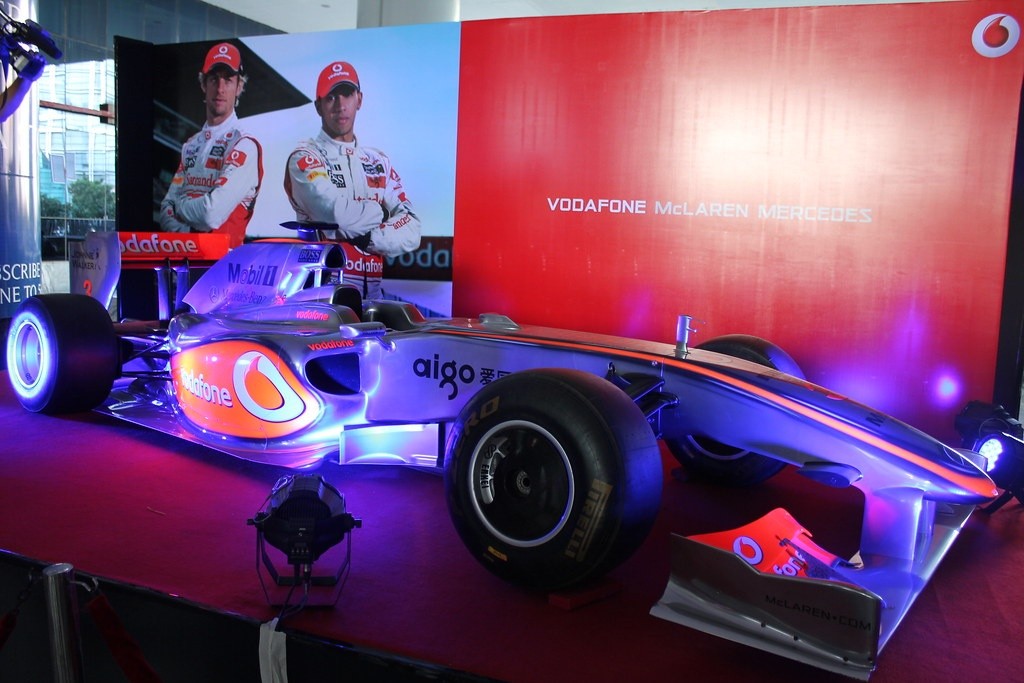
[0,50,47,123]
[283,61,422,300]
[159,43,264,253]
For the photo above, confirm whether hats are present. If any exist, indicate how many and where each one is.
[203,43,241,77]
[316,62,359,99]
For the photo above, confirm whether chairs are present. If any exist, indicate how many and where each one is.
[332,284,363,323]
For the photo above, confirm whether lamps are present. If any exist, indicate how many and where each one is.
[245,473,363,612]
